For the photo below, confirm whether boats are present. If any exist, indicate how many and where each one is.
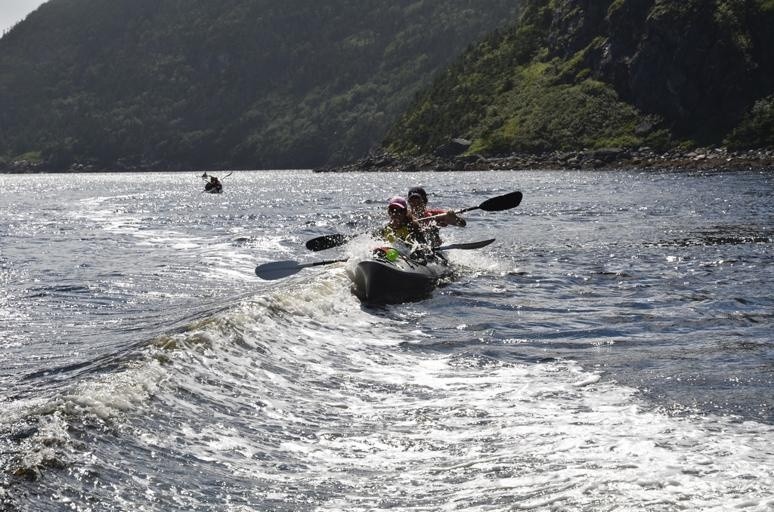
[205,182,222,193]
[345,248,453,308]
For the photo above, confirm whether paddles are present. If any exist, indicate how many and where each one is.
[306,192,523,252]
[254,238,496,280]
[219,170,234,180]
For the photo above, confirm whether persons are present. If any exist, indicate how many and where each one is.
[370,186,467,266]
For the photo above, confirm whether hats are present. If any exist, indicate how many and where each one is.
[388,196,407,209]
[408,186,427,200]
